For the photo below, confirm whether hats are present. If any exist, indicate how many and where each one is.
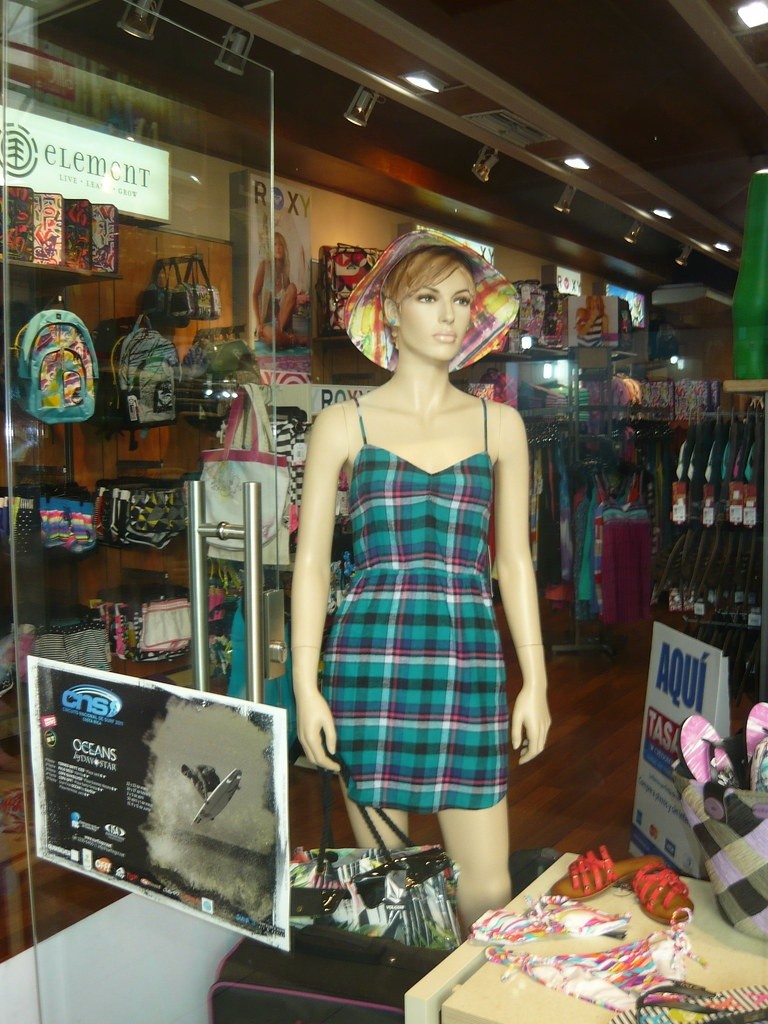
[344,231,520,375]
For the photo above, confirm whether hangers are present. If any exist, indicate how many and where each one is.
[95,571,192,607]
[41,486,90,506]
[96,460,183,491]
[588,403,759,425]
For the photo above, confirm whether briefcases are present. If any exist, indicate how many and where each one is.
[209,925,448,1024]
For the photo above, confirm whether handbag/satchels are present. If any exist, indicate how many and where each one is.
[200,383,288,550]
[671,737,768,941]
[141,257,191,328]
[289,753,462,950]
[314,242,565,415]
[172,255,221,320]
[105,313,182,427]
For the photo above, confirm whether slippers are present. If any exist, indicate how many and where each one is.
[610,985,768,1024]
[679,702,768,787]
[630,860,695,923]
[550,845,666,901]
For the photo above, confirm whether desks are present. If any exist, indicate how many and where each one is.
[404,852,768,1024]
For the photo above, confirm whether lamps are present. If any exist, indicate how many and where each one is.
[473,145,499,182]
[675,245,693,268]
[214,26,254,76]
[623,221,643,245]
[117,0,163,42]
[344,85,379,128]
[553,184,577,215]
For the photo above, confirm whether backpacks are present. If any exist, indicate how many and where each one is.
[11,295,99,425]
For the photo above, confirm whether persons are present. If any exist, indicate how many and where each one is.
[253,232,310,351]
[291,228,552,941]
[575,295,609,347]
